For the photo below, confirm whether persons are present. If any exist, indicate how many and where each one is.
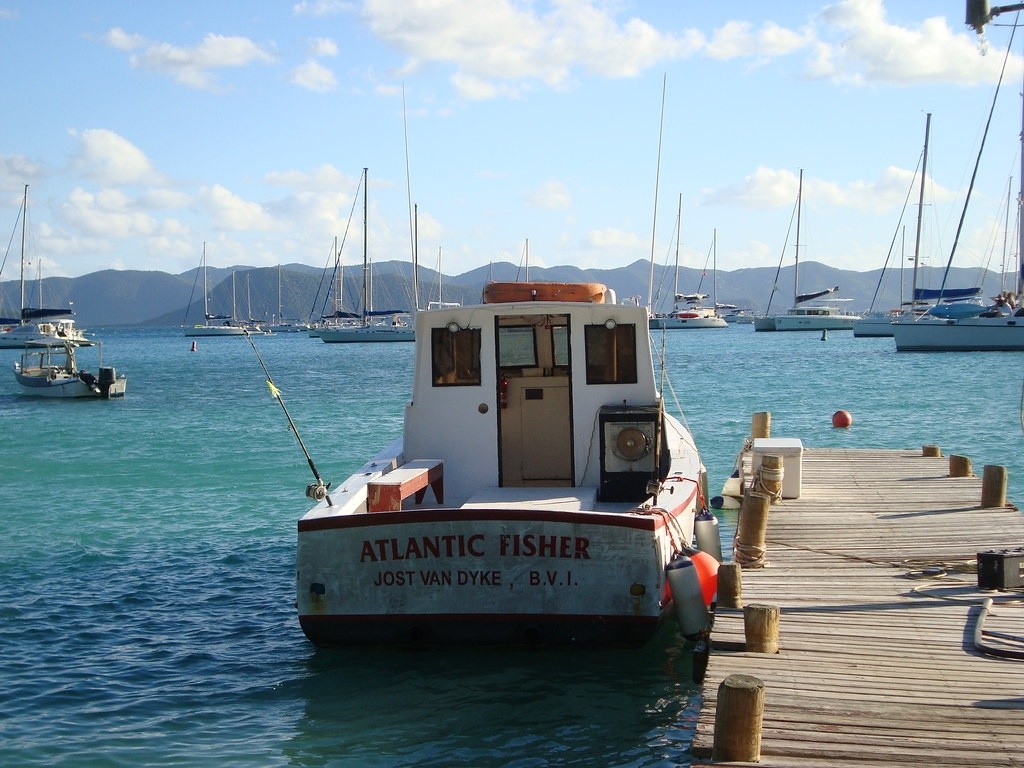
[1005,293,1017,309]
[996,295,1003,308]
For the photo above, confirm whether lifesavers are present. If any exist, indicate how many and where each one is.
[679,312,698,318]
[482,281,608,301]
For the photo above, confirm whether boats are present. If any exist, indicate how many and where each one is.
[290,280,715,662]
[14,332,127,401]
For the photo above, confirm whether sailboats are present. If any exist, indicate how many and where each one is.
[754,168,866,331]
[709,228,756,324]
[0,180,96,351]
[180,240,245,339]
[851,111,963,336]
[889,1,1024,354]
[225,168,540,344]
[647,190,728,330]
